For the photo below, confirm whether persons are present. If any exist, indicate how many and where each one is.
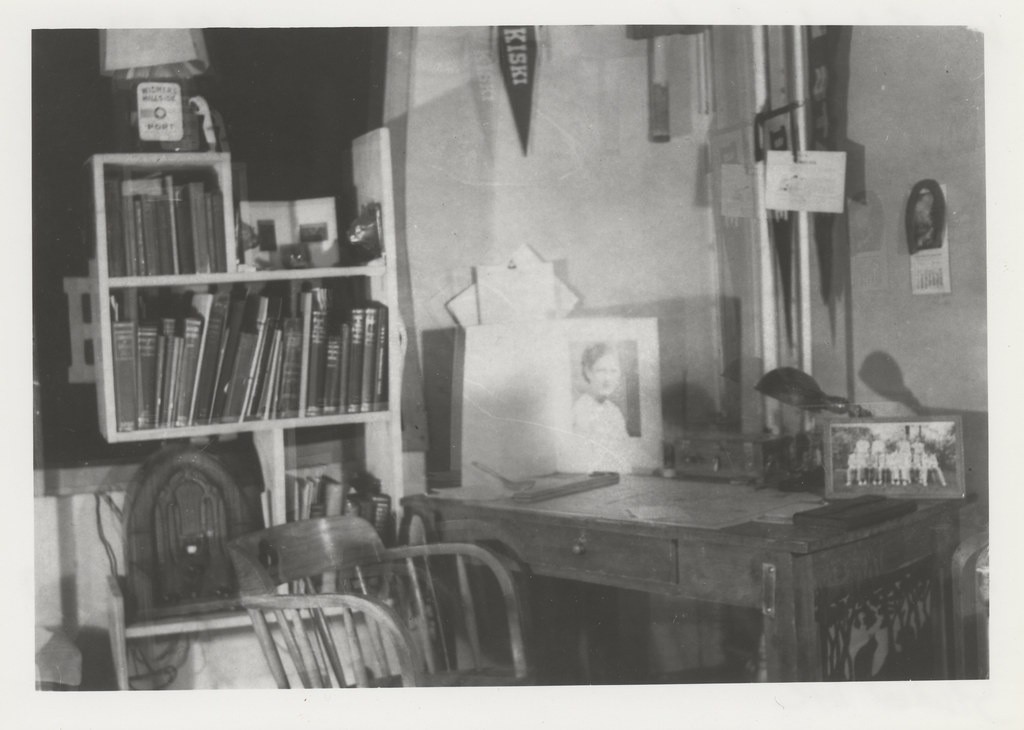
[570,342,630,441]
[842,432,946,488]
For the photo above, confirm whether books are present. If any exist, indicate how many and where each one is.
[283,467,401,606]
[103,174,390,434]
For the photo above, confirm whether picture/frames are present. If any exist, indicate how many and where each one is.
[562,316,664,474]
[824,416,968,498]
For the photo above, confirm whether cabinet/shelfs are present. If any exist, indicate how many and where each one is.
[84,151,401,690]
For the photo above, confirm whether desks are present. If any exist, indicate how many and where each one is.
[397,472,980,680]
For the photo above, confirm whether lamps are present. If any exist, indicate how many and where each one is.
[751,365,875,498]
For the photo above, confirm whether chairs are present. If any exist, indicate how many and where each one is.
[228,516,527,687]
